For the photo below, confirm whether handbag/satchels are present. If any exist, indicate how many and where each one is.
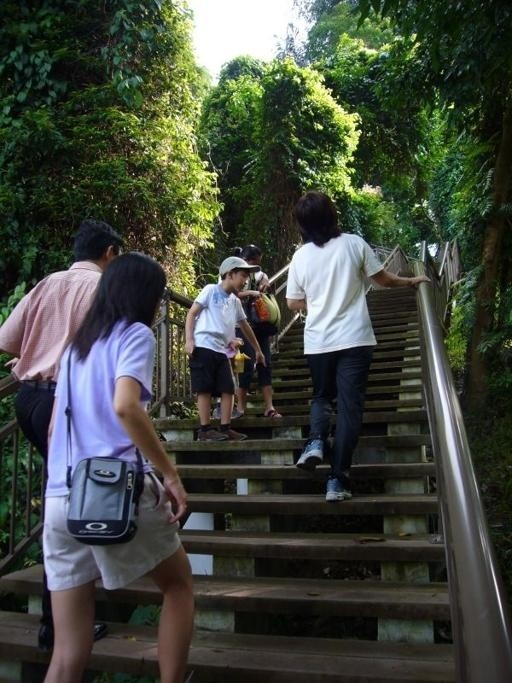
[66,457,144,546]
[249,293,281,336]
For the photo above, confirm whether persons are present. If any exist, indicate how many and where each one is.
[1,215,130,654]
[41,246,199,682]
[184,256,267,443]
[282,181,434,504]
[227,242,282,421]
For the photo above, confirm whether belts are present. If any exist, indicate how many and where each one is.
[19,379,55,390]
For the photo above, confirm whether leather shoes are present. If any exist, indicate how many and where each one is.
[37,623,107,652]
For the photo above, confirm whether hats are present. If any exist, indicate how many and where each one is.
[219,257,260,277]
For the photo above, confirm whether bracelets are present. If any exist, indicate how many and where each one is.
[411,277,415,287]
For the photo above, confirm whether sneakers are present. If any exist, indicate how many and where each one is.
[196,404,282,443]
[325,476,353,501]
[296,440,324,471]
